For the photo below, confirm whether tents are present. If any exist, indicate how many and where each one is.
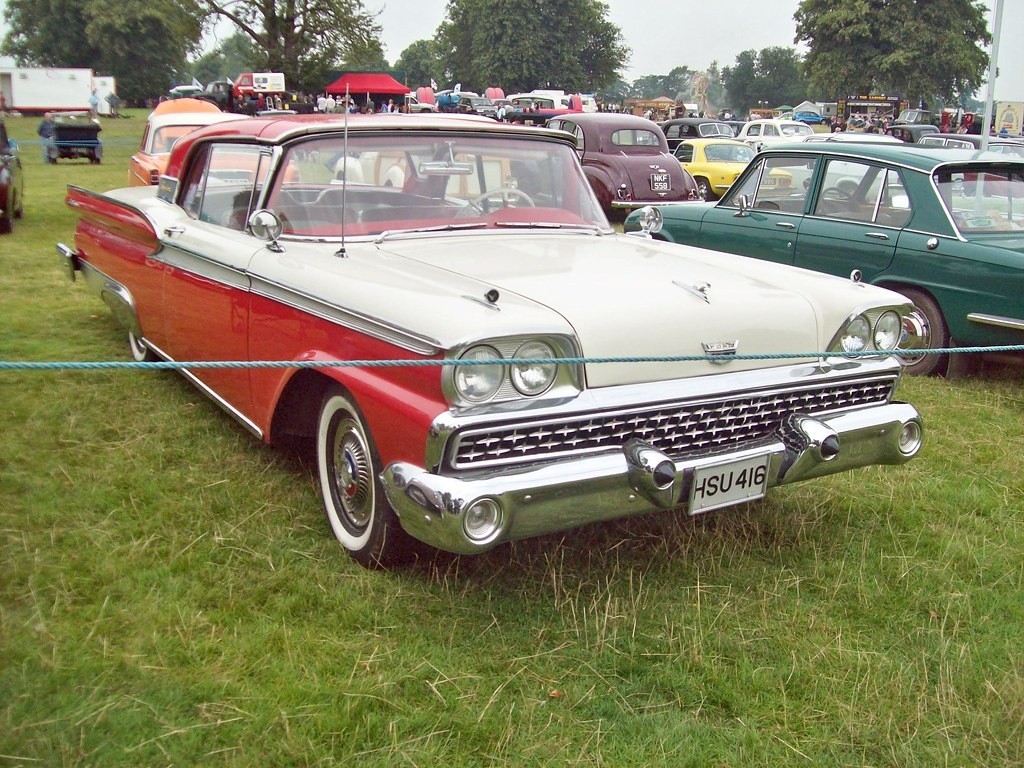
[325,73,410,106]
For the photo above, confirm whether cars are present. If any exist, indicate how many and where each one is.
[800,132,903,146]
[490,99,510,106]
[126,99,253,186]
[673,138,792,198]
[736,119,814,146]
[54,118,923,572]
[726,120,761,138]
[0,120,25,233]
[612,141,1024,376]
[661,117,734,156]
[774,112,792,121]
[539,113,703,213]
[405,95,435,114]
[506,94,555,113]
[47,110,103,165]
[916,133,1024,161]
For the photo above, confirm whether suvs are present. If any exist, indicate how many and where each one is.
[793,110,829,126]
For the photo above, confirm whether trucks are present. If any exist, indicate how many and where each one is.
[192,81,316,112]
[232,73,285,101]
[895,107,914,129]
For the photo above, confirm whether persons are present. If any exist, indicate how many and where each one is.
[233,92,407,118]
[90,89,99,118]
[0,91,9,117]
[596,102,772,121]
[37,112,54,164]
[830,115,1009,139]
[106,92,119,114]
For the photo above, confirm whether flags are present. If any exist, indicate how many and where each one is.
[192,77,203,89]
[430,78,438,91]
[227,77,233,84]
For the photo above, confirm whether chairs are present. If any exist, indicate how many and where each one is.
[224,189,477,230]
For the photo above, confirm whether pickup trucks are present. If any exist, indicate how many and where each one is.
[440,96,497,117]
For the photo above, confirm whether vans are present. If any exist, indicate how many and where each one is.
[560,95,598,113]
[169,84,203,97]
[885,124,940,143]
